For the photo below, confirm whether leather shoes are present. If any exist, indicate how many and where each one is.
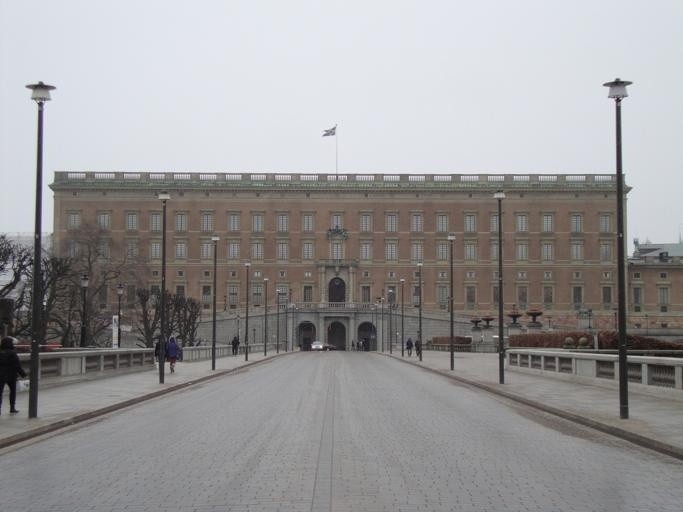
[10,409,20,414]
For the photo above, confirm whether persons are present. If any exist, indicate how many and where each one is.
[415,340,419,356]
[351,340,359,352]
[232,337,240,355]
[155,336,179,373]
[0,336,27,413]
[407,337,413,356]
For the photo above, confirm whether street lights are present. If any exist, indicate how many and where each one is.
[416,258,423,361]
[208,233,295,371]
[369,277,404,358]
[446,232,457,374]
[79,273,88,349]
[603,75,636,418]
[115,284,123,349]
[492,189,506,387]
[547,307,650,337]
[155,188,169,385]
[24,77,57,424]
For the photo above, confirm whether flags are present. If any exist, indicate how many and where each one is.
[323,126,336,136]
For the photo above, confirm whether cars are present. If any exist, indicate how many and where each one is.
[309,341,323,351]
[322,343,336,351]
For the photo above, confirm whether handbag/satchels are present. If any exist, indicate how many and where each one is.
[16,379,30,392]
